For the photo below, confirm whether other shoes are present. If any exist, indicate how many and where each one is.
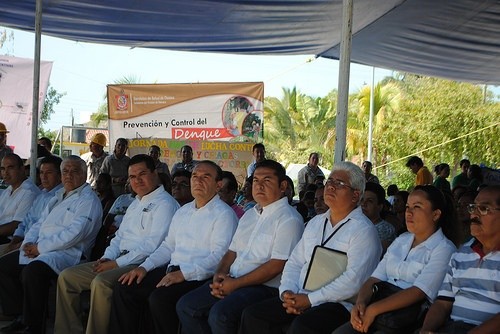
[13,325,34,334]
[0,319,25,333]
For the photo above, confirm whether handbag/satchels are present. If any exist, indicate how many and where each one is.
[368,281,430,333]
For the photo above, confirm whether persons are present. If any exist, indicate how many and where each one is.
[334,185,456,334]
[149,145,198,205]
[53,154,180,334]
[0,155,103,334]
[0,153,40,245]
[176,160,304,334]
[218,144,266,219]
[241,161,383,334]
[360,156,433,252]
[434,159,485,246]
[0,156,67,257]
[282,153,329,224]
[249,120,258,143]
[418,184,500,334]
[0,123,13,158]
[110,160,238,334]
[38,137,51,152]
[81,133,135,260]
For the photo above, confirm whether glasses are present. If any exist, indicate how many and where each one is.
[326,179,355,192]
[171,181,190,188]
[466,204,500,215]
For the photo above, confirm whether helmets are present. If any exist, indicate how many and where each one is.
[91,133,107,147]
[0,122,10,133]
